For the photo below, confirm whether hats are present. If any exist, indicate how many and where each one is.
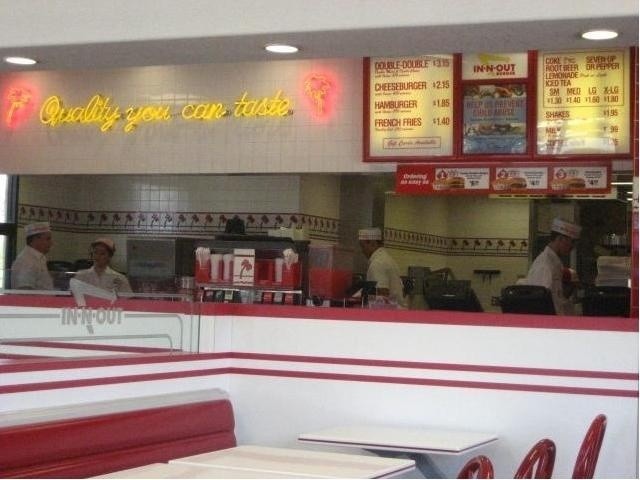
[90,237,116,254]
[358,228,383,242]
[549,217,584,242]
[24,222,52,239]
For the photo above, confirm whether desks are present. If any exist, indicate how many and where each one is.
[169,444,419,478]
[299,420,498,479]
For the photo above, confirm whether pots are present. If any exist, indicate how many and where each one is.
[601,230,626,248]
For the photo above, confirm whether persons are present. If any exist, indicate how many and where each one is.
[358,227,404,307]
[75,238,135,298]
[514,271,527,285]
[12,222,55,291]
[528,218,582,315]
[558,255,580,283]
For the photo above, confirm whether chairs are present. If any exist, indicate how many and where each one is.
[570,414,607,479]
